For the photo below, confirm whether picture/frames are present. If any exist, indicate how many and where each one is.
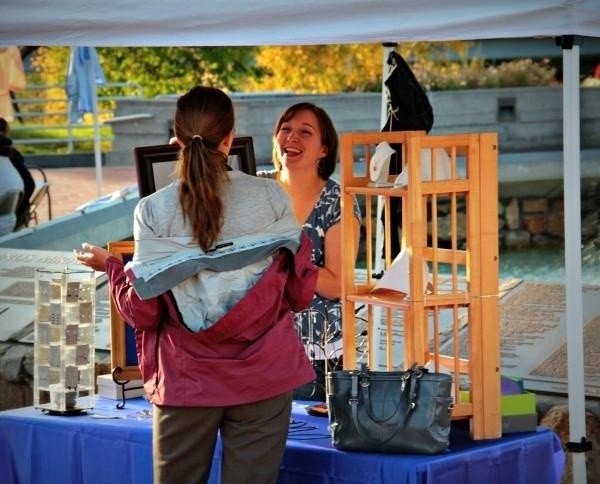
[131,135,258,197]
[106,239,146,382]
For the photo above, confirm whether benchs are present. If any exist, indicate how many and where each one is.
[24,164,53,228]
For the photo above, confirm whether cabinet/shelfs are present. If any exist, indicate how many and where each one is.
[338,130,503,441]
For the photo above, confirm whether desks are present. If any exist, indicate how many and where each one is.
[0,393,555,483]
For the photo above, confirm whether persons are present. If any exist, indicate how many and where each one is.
[73,86,319,484]
[257,103,363,401]
[0,117,35,237]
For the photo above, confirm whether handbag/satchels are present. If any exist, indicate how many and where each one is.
[327,368,453,454]
